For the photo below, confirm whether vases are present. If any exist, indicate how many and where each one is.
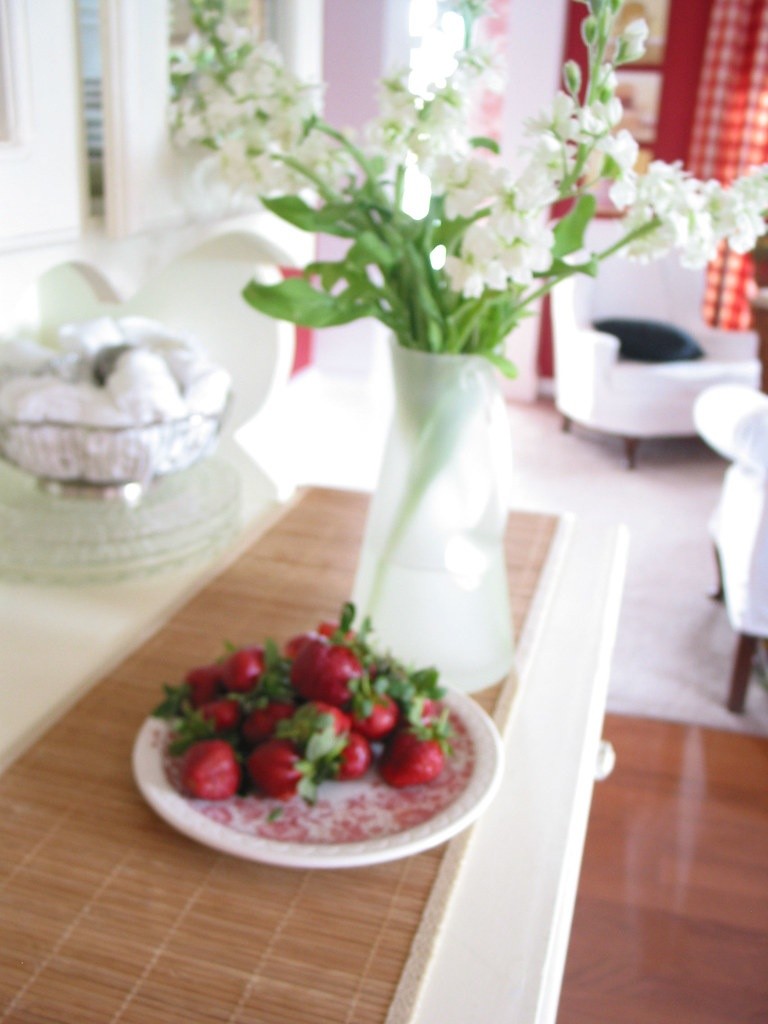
[347,334,519,690]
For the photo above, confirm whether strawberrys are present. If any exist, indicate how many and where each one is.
[174,621,446,802]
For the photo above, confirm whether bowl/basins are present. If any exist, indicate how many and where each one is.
[0,392,233,501]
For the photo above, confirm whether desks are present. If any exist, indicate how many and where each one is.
[0,484,627,1024]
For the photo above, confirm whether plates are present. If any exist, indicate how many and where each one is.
[132,686,505,870]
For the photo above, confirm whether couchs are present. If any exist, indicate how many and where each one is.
[692,381,768,713]
[540,213,765,469]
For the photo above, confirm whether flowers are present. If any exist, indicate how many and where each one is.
[165,0,768,379]
